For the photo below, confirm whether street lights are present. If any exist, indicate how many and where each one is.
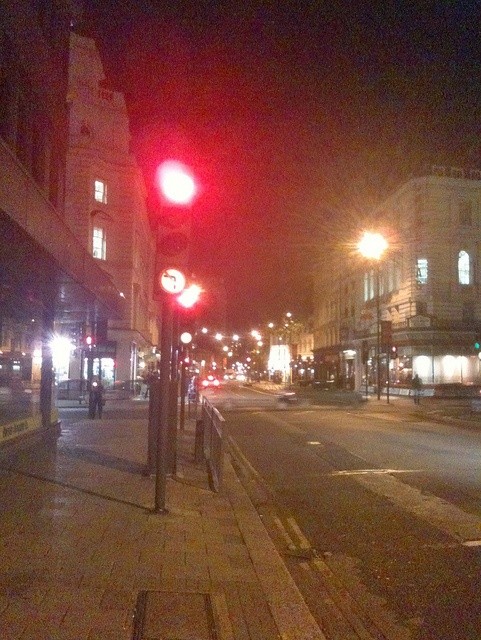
[179,330,193,432]
[360,231,389,403]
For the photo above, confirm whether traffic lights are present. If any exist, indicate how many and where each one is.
[152,159,196,304]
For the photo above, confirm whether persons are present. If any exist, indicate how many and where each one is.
[414,374,423,405]
[92,381,105,420]
